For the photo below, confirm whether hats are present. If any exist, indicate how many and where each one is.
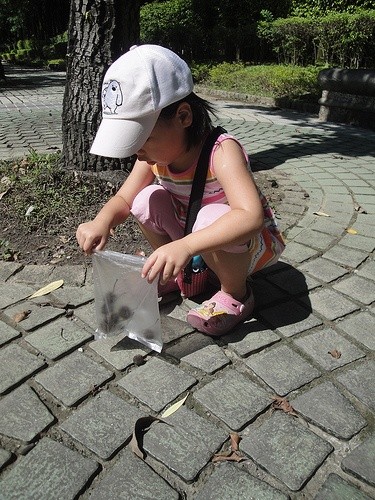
[89,44,193,158]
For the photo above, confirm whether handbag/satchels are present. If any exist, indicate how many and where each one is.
[178,267,209,299]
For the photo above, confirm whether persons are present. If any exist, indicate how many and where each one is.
[74,44,286,336]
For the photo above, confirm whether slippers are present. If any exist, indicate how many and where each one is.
[158,276,181,296]
[187,282,255,335]
